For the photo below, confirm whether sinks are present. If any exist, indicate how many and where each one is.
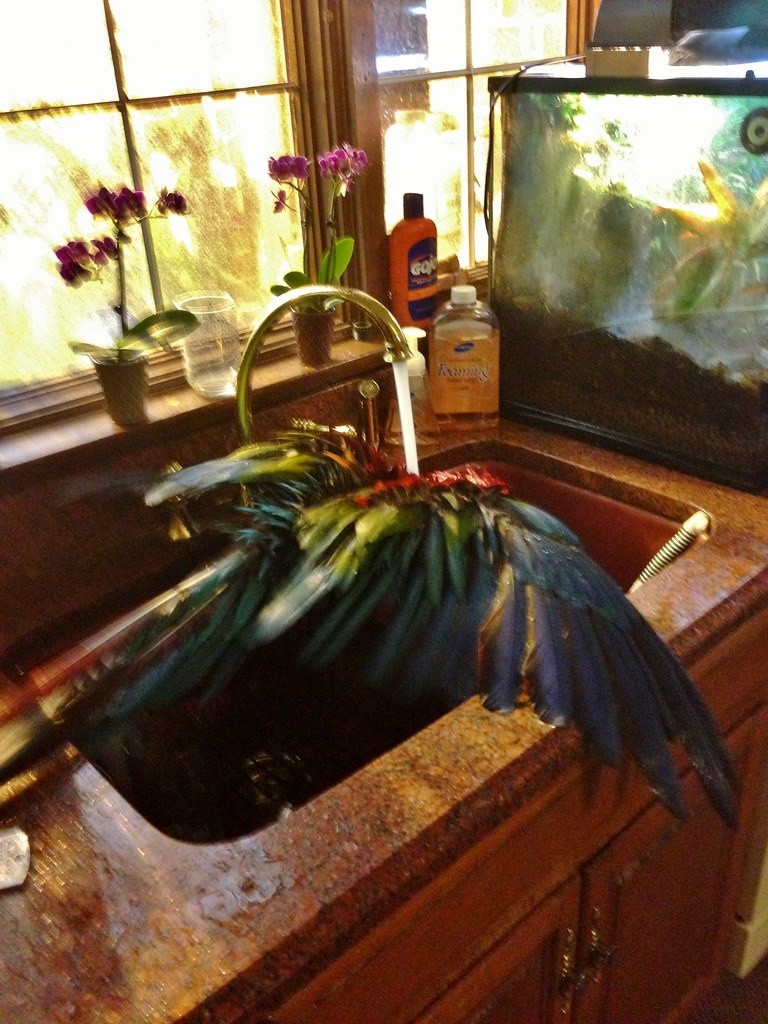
[239,285,413,443]
[38,435,712,846]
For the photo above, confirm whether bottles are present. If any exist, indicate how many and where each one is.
[387,193,438,334]
[427,284,501,432]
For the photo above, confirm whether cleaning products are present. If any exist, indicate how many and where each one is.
[390,193,436,326]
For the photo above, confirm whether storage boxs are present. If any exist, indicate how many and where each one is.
[488,64,768,495]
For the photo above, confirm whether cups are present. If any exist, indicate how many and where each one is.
[172,291,239,398]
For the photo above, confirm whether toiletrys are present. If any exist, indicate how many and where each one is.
[429,285,501,431]
[384,327,441,447]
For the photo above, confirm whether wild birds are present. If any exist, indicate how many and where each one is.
[1,418,742,848]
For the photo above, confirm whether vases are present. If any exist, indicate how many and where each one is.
[92,355,152,425]
[290,309,337,363]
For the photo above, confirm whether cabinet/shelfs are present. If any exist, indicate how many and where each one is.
[245,613,768,1024]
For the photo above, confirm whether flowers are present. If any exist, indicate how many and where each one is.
[267,144,370,310]
[53,182,200,361]
